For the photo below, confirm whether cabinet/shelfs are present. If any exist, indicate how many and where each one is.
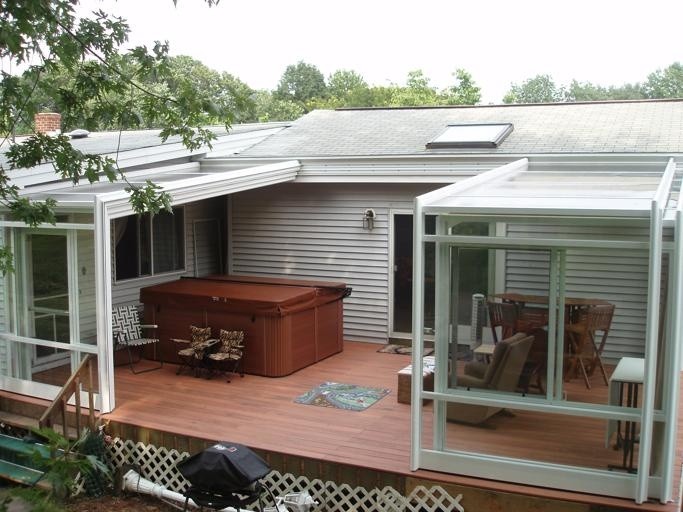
[140,274,352,378]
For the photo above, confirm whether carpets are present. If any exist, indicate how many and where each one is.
[376,343,473,362]
[292,380,391,412]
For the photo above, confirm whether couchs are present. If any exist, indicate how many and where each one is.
[446,333,535,429]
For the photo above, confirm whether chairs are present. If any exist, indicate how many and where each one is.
[169,326,211,378]
[486,300,535,348]
[209,329,245,383]
[565,305,615,388]
[112,306,162,374]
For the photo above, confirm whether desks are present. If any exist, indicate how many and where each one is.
[486,293,615,380]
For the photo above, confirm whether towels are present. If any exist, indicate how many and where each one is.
[605,356,646,449]
